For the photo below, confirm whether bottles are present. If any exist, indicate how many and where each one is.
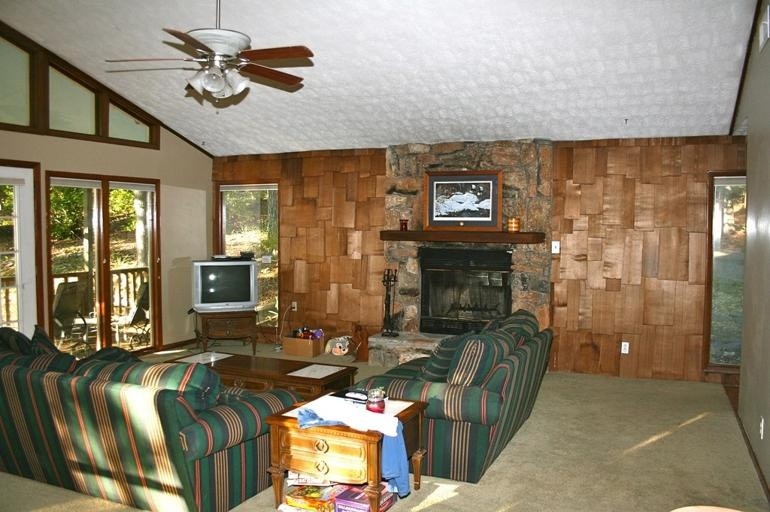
[366,389,385,413]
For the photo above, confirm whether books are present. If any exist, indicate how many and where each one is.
[282,469,399,512]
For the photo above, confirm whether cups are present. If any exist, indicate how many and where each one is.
[507,217,520,232]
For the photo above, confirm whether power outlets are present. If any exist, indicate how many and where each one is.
[621,341,630,354]
[551,241,561,254]
[291,302,298,312]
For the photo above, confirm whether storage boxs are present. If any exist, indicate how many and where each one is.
[283,336,326,357]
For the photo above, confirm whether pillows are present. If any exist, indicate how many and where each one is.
[1,323,225,410]
[414,310,540,386]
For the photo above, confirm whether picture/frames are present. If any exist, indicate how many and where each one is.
[421,171,504,232]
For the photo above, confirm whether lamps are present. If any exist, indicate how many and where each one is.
[186,64,250,98]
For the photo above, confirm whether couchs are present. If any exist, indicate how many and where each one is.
[352,328,553,483]
[0,364,305,512]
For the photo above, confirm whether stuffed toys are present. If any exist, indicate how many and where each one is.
[330,336,350,357]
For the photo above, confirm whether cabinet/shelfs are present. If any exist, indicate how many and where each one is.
[196,311,258,355]
[266,390,429,512]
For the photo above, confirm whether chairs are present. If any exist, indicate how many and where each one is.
[111,283,150,349]
[52,280,93,355]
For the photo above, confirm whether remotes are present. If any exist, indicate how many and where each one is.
[240,252,254,257]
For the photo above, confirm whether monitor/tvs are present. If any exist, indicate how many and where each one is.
[192,260,256,313]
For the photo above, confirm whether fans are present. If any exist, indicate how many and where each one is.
[106,1,314,87]
